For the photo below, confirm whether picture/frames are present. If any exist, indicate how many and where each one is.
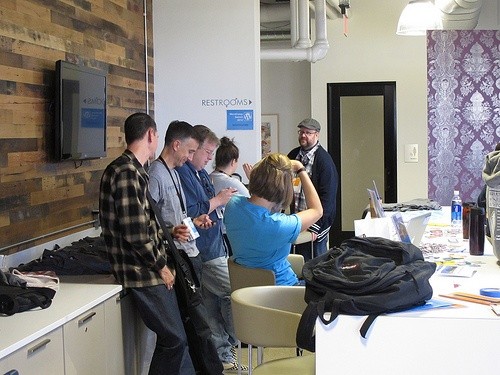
[261,114,280,159]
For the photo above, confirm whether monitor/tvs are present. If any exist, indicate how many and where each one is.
[51,60,107,161]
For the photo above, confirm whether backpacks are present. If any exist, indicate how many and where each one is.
[296,236,439,353]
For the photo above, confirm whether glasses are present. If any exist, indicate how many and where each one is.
[298,131,317,137]
[200,146,214,158]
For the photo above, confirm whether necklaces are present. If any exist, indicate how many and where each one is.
[215,167,232,178]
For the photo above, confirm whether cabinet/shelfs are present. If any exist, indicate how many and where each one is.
[0,273,158,375]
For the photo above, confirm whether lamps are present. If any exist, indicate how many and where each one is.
[396,0,443,36]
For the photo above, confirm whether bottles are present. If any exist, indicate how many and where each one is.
[450,190,461,228]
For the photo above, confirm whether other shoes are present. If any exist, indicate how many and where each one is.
[222,361,249,372]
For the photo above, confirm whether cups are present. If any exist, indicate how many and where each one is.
[463,201,476,239]
[469,207,485,255]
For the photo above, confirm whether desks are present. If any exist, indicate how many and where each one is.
[315,206,500,375]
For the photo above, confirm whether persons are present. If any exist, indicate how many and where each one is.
[179,123,239,371]
[148,119,237,375]
[98,111,201,373]
[208,137,255,258]
[289,117,338,266]
[221,153,324,286]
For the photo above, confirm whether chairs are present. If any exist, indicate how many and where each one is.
[231,286,316,375]
[292,231,317,259]
[227,254,304,292]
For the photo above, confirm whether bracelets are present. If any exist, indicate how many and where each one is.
[296,167,306,174]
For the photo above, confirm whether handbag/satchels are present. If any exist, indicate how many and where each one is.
[0,282,56,316]
[167,248,203,308]
[11,268,60,285]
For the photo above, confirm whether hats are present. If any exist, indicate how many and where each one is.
[297,119,321,132]
